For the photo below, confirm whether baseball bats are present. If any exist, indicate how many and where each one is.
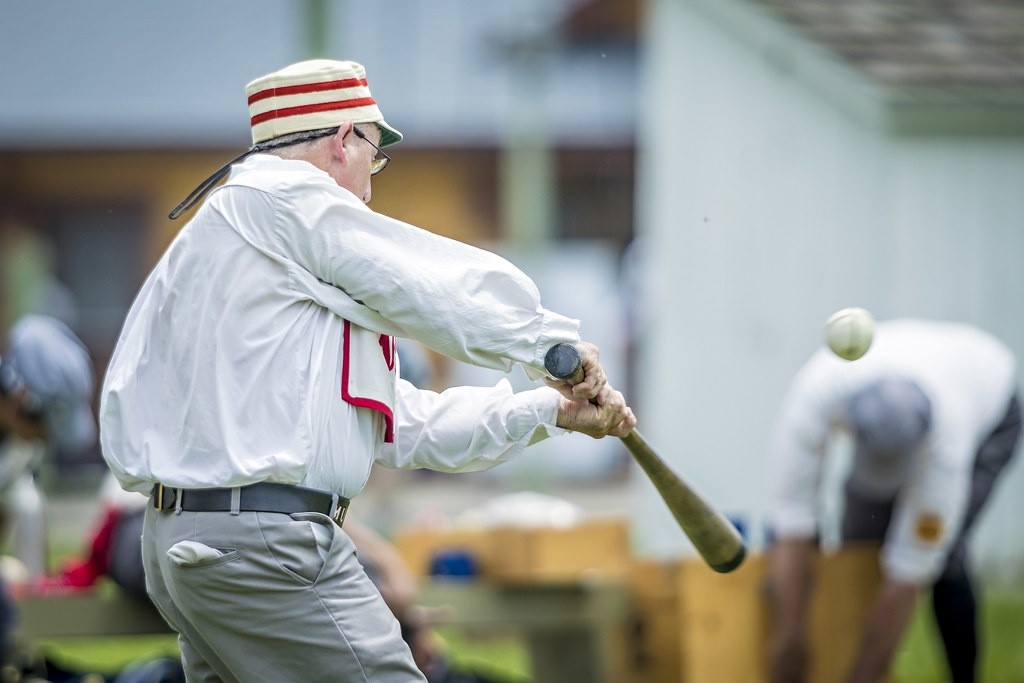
[543,342,751,574]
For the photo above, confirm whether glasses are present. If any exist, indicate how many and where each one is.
[327,125,391,177]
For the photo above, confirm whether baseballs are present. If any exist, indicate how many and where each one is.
[823,304,875,360]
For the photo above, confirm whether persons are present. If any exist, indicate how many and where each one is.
[97,58,637,683]
[45,465,450,682]
[1,315,95,601]
[759,318,1024,683]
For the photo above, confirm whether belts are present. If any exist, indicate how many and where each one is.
[150,481,349,526]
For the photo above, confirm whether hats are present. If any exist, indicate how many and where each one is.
[246,58,403,147]
[850,380,932,452]
[3,317,98,455]
